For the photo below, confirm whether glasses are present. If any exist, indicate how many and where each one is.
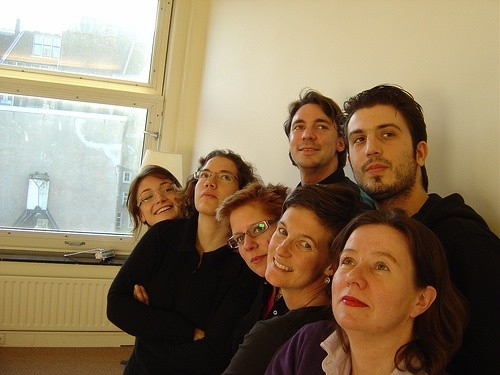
[137,185,181,208]
[227,217,279,249]
[194,169,241,185]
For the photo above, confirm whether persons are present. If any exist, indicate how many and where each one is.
[221,182,377,375]
[284,85,362,198]
[338,85,500,374]
[216,177,293,356]
[127,165,187,243]
[264,207,466,375]
[106,149,272,375]
